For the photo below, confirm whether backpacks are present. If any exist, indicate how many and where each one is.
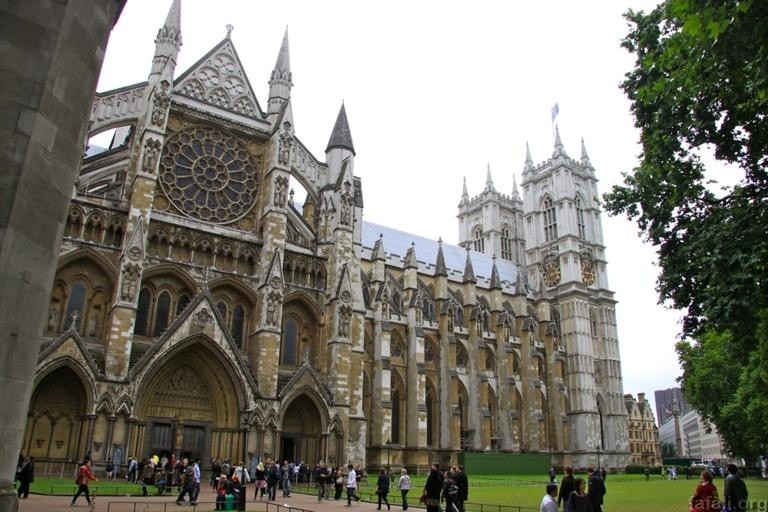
[446,479,458,496]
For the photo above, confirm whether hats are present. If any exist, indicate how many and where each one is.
[256,463,264,471]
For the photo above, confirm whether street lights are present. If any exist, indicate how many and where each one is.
[236,397,253,511]
[596,442,602,470]
[720,448,725,461]
[385,438,392,489]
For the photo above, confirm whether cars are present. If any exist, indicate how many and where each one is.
[691,458,731,476]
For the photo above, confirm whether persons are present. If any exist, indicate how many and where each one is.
[84,450,92,466]
[539,466,605,510]
[17,456,34,498]
[107,452,202,505]
[375,469,390,510]
[209,450,360,510]
[70,460,99,506]
[398,469,410,511]
[14,448,25,491]
[422,462,468,512]
[645,458,754,511]
